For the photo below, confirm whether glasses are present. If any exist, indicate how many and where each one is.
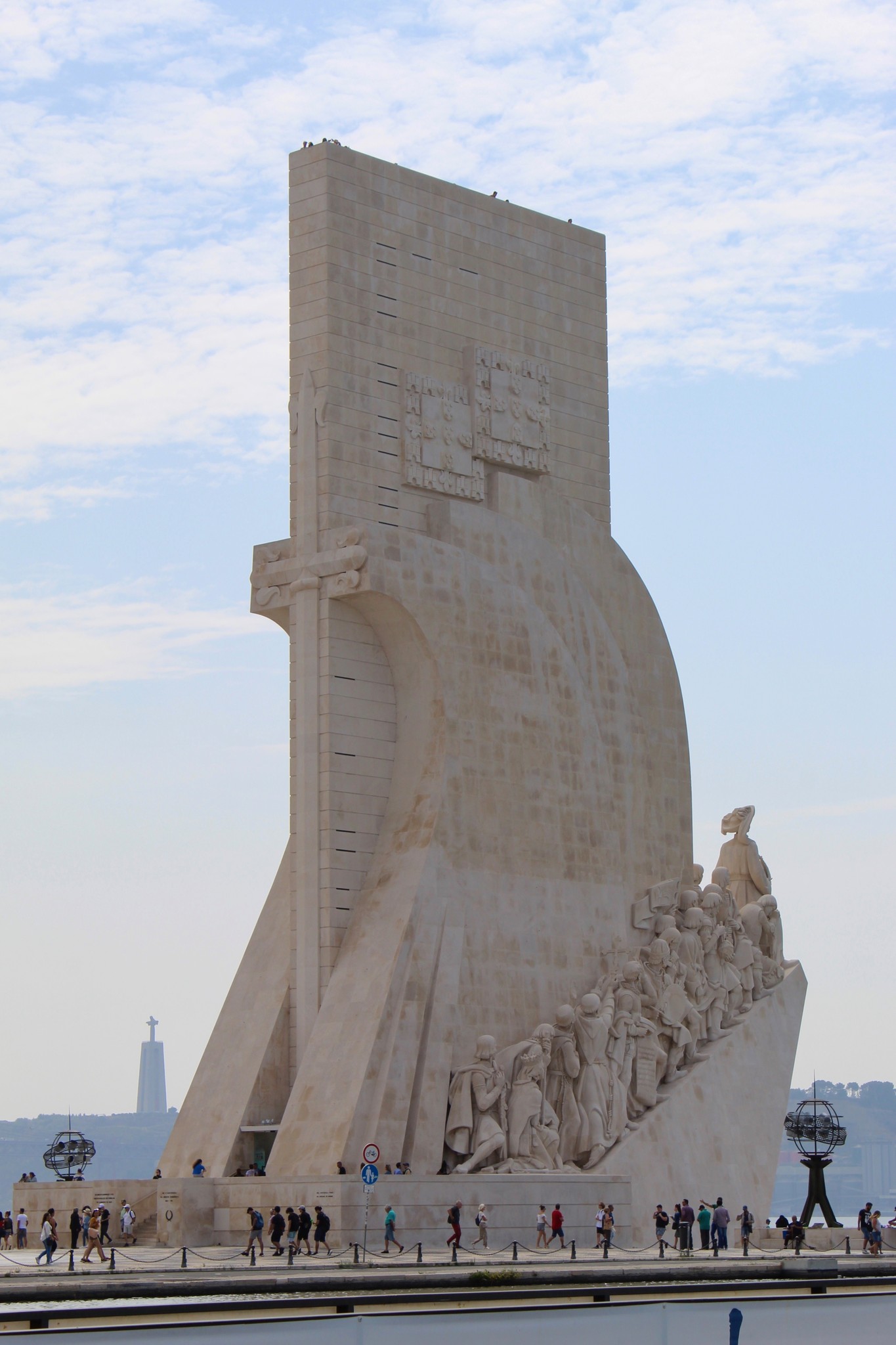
[94,1209,99,1211]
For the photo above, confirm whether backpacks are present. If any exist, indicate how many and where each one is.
[661,1211,669,1226]
[475,1212,482,1226]
[256,1168,266,1176]
[447,1206,456,1223]
[303,1212,312,1229]
[254,1211,264,1228]
[319,1213,330,1233]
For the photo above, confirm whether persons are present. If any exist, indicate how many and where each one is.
[436,809,799,1175]
[192,1159,206,1177]
[857,1202,896,1259]
[535,1205,552,1248]
[153,1169,162,1180]
[652,1197,755,1250]
[544,1203,568,1249]
[471,1204,491,1249]
[72,1168,85,1181]
[337,1161,412,1175]
[234,1163,266,1177]
[0,1199,138,1265]
[19,1172,37,1183]
[763,1215,806,1254]
[381,1204,405,1254]
[591,1202,616,1249]
[239,1205,334,1257]
[445,1200,462,1249]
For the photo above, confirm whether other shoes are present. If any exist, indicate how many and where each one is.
[239,1242,883,1257]
[0,1237,137,1266]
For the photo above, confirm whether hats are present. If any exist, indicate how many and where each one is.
[403,1162,409,1166]
[73,1198,130,1214]
[698,1205,705,1210]
[297,1205,305,1209]
[766,1219,770,1222]
[742,1205,747,1209]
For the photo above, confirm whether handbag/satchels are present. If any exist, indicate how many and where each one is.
[604,1219,612,1231]
[483,1223,488,1229]
[46,1240,54,1246]
[77,1176,82,1181]
[672,1223,678,1230]
[87,1227,99,1239]
[748,1225,752,1233]
